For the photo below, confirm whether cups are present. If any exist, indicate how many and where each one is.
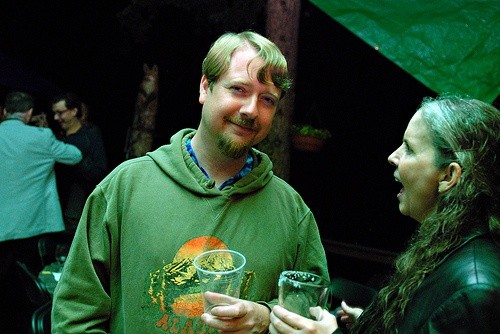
[279,271,332,321]
[193,249,246,320]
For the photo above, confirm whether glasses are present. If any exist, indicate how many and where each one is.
[51,110,67,116]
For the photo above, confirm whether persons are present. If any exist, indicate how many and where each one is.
[49,32,333,334]
[52,94,105,246]
[0,91,82,334]
[269,95,500,334]
